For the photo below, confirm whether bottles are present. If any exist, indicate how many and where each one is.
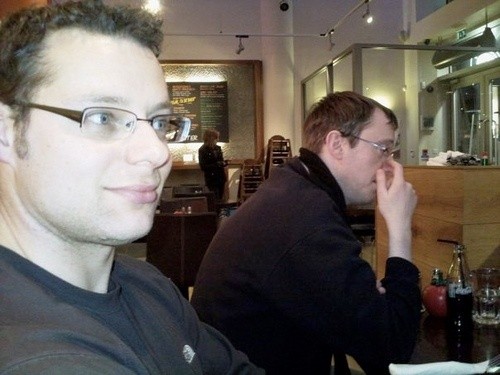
[446,245,473,350]
[421,150,428,161]
[422,268,447,318]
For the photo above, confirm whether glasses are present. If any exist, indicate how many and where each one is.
[340,129,401,162]
[12,97,192,145]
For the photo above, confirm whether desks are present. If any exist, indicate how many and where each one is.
[144,211,220,304]
[371,164,500,299]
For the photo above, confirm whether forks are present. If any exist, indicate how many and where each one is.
[486,355,500,370]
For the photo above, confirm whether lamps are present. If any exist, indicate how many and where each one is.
[233,35,249,55]
[479,4,497,51]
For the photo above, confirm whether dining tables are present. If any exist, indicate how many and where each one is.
[350,284,500,375]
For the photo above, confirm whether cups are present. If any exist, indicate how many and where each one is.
[471,267,500,325]
[480,151,490,166]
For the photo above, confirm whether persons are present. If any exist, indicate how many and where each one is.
[191,91,423,375]
[0,0,268,375]
[198,129,226,214]
